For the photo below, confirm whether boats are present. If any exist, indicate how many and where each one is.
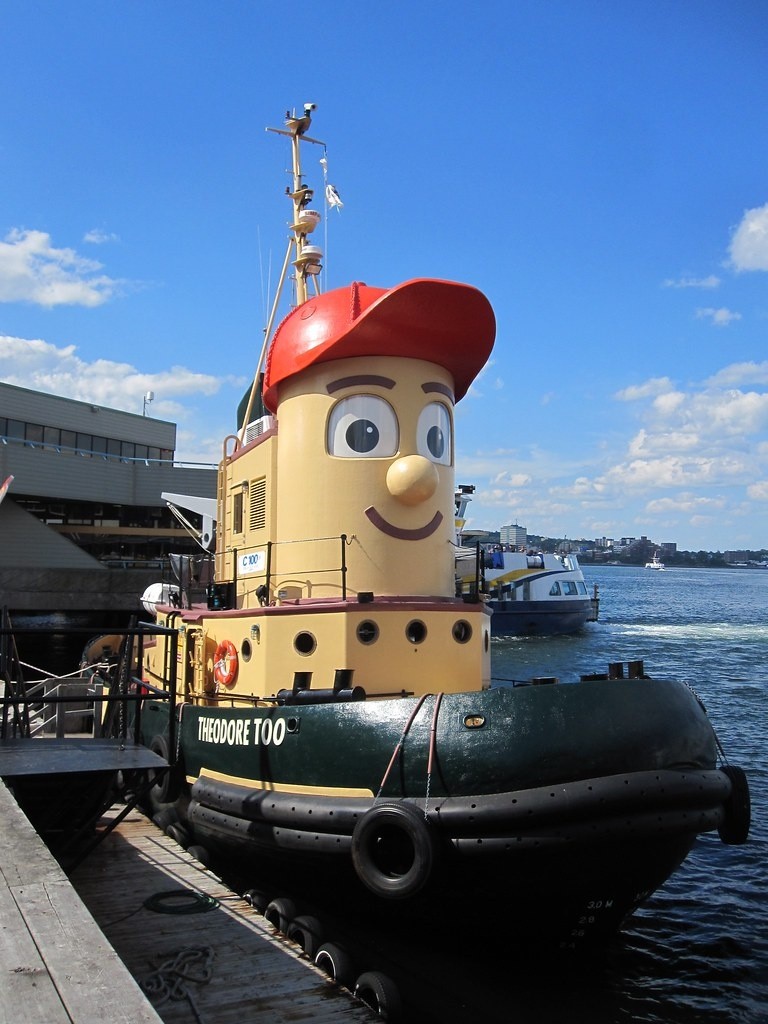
[80,96,755,1021]
[643,549,666,569]
[458,544,601,637]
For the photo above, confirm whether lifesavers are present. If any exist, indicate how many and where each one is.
[350,800,440,901]
[242,888,267,915]
[313,943,353,987]
[352,971,398,1018]
[263,897,296,940]
[212,639,239,687]
[186,845,210,866]
[715,763,753,848]
[151,812,169,832]
[286,914,324,963]
[146,734,185,805]
[164,821,189,850]
